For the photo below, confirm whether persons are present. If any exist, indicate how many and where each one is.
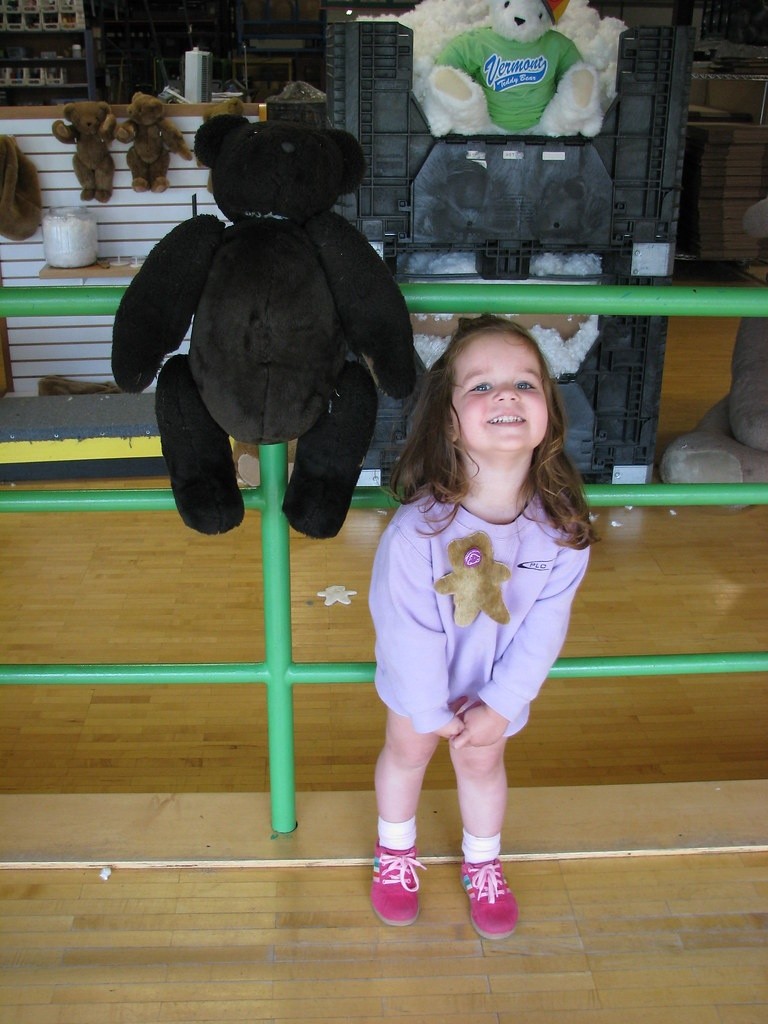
[368,313,595,941]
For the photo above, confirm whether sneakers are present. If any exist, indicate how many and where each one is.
[461,856,518,939]
[371,836,427,927]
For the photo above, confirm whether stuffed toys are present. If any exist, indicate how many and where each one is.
[51,101,115,204]
[110,115,416,537]
[1,134,43,242]
[426,1,605,138]
[659,195,767,510]
[112,92,193,192]
[196,98,244,193]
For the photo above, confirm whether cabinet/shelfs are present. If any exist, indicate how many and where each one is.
[599,0,768,270]
[0,29,96,103]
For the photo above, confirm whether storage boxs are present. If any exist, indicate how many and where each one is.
[321,20,696,277]
[268,101,326,126]
[355,277,676,486]
[0,0,86,86]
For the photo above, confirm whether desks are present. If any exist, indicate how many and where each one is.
[38,257,140,280]
[232,58,315,84]
